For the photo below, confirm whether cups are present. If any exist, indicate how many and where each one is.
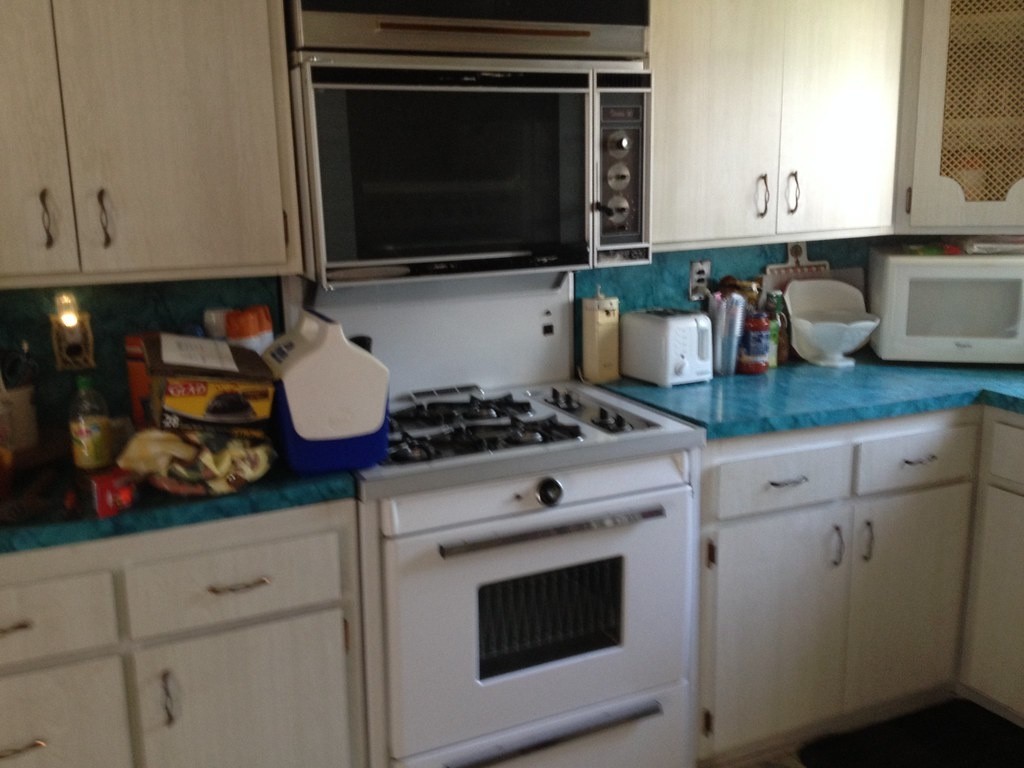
[708,293,747,375]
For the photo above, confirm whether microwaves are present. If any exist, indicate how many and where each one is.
[867,247,1023,364]
[285,49,652,291]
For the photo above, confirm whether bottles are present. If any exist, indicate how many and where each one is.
[737,312,769,374]
[70,377,112,489]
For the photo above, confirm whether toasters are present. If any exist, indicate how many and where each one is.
[621,307,714,388]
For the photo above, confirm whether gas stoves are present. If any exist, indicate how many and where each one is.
[312,273,705,499]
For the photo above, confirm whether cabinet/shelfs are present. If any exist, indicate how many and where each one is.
[636,1,895,255]
[954,405,1024,729]
[896,0,1023,239]
[0,498,372,768]
[0,1,304,289]
[693,401,984,768]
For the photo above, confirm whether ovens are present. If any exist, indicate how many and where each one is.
[380,451,699,768]
